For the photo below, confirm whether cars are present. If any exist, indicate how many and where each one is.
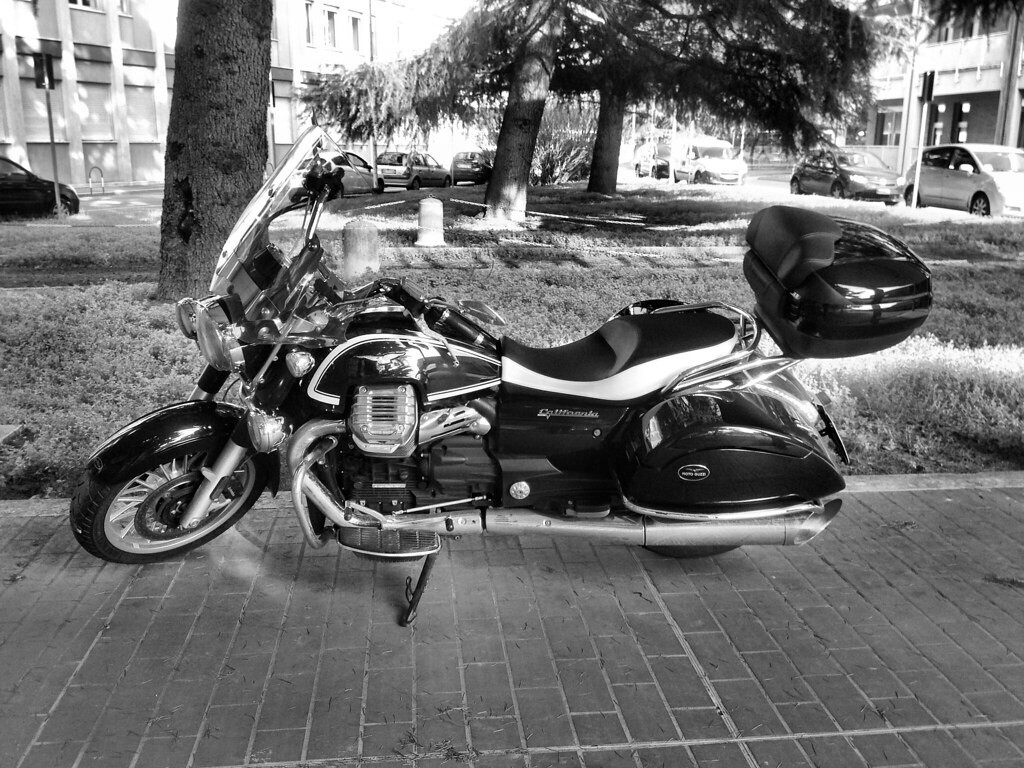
[288,150,385,203]
[632,142,670,180]
[790,148,907,206]
[449,151,490,185]
[377,151,452,191]
[674,134,747,185]
[902,144,1024,216]
[0,156,80,219]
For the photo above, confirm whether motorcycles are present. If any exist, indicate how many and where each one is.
[69,124,933,628]
